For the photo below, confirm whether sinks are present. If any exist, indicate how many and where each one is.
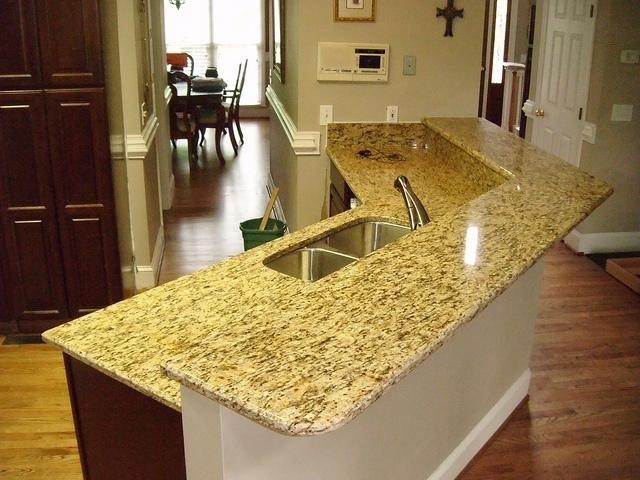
[265,248,360,283]
[326,221,412,256]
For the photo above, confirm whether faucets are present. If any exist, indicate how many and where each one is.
[393,174,431,231]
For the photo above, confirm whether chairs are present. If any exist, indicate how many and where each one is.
[167,52,248,172]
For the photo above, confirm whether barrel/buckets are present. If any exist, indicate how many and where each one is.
[239,217,287,251]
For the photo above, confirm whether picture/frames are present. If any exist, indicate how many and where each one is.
[271,0,285,84]
[333,0,377,22]
